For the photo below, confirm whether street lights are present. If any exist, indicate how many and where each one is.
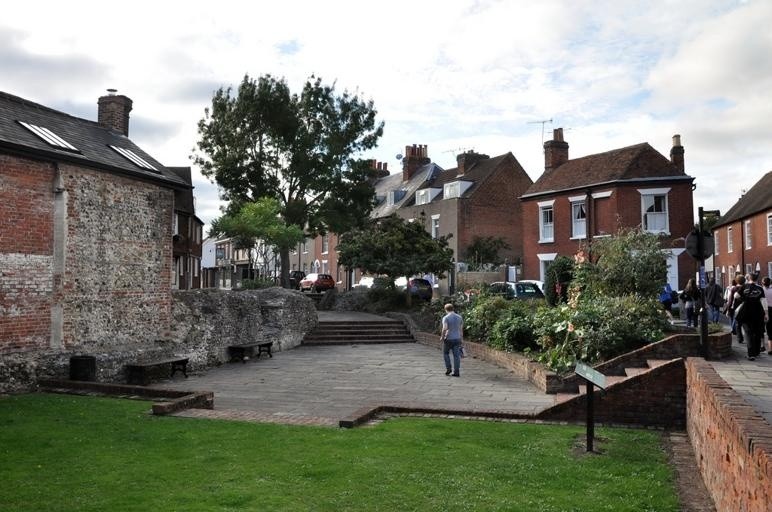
[418,209,426,228]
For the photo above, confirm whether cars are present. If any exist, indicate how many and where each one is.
[276,269,334,293]
[352,277,432,302]
[490,279,545,298]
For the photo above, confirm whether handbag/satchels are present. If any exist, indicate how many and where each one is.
[458,345,468,359]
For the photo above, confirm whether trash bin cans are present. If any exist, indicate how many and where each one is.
[70,356,97,391]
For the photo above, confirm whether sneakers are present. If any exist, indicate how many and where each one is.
[445,368,460,377]
[730,330,772,361]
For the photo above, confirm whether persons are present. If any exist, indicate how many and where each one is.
[655,271,771,361]
[437,302,465,378]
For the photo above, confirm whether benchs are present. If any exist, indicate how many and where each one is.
[123,356,189,385]
[228,341,274,364]
